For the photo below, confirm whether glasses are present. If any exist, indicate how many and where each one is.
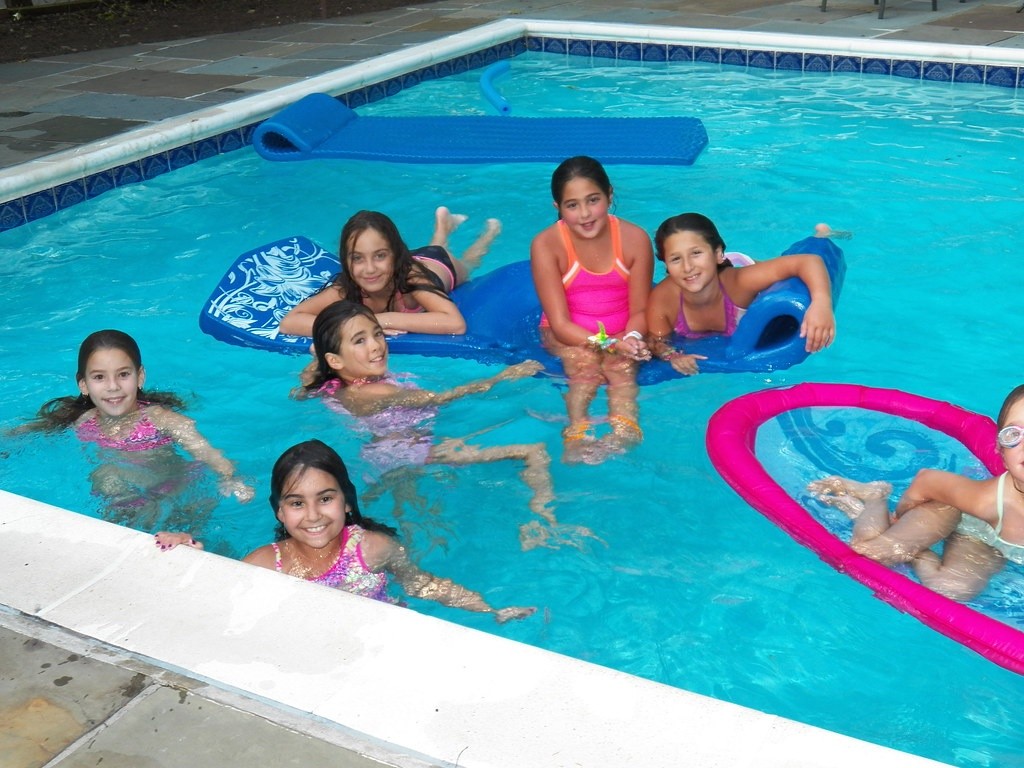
[996,426,1024,450]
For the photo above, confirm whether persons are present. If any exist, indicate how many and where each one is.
[154,436,538,622]
[34,332,256,503]
[299,302,547,467]
[529,153,654,361]
[807,385,1024,601]
[643,211,832,376]
[276,203,500,338]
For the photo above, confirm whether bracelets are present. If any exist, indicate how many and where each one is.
[623,330,644,341]
[584,315,620,355]
